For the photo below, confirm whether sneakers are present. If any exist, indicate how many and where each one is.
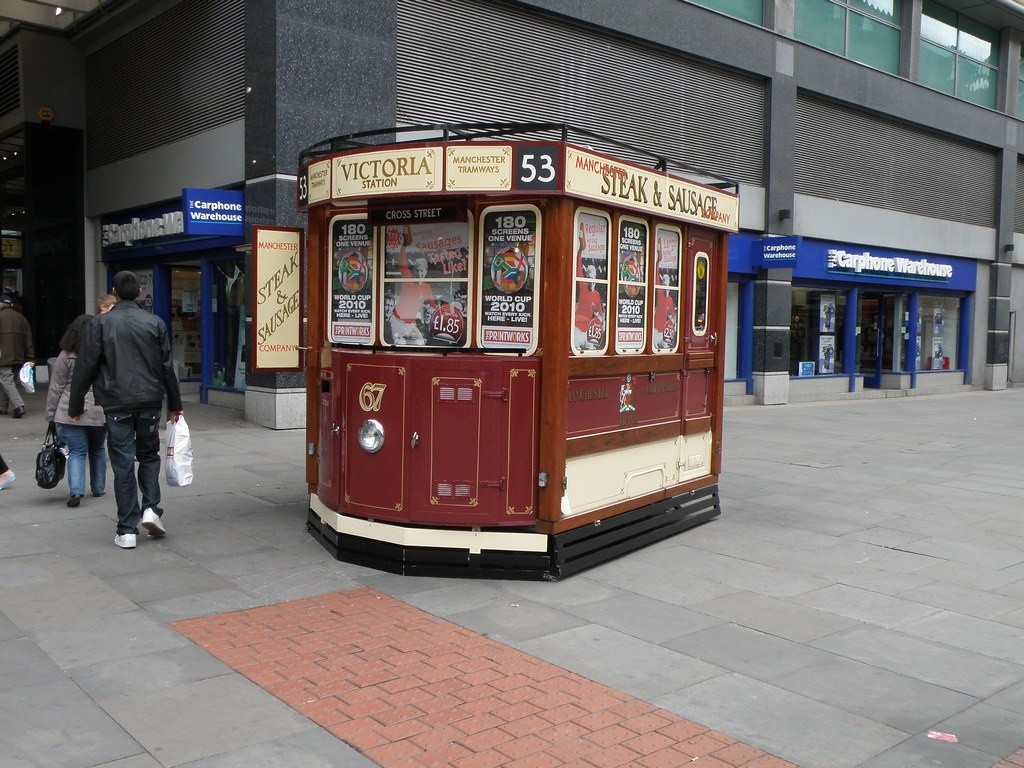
[141,508,165,536]
[114,534,138,547]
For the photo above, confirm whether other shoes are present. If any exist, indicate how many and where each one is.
[0,472,14,491]
[66,495,80,508]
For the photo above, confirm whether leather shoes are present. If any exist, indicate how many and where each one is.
[12,406,25,419]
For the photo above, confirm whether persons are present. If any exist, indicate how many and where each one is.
[382,292,396,345]
[0,293,37,418]
[389,227,438,347]
[574,230,606,350]
[0,454,16,492]
[417,306,438,348]
[41,314,110,509]
[459,289,467,316]
[94,295,138,464]
[450,292,465,318]
[653,244,677,350]
[66,271,185,550]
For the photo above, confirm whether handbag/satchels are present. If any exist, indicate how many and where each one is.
[19,362,35,394]
[165,414,194,486]
[36,421,66,490]
